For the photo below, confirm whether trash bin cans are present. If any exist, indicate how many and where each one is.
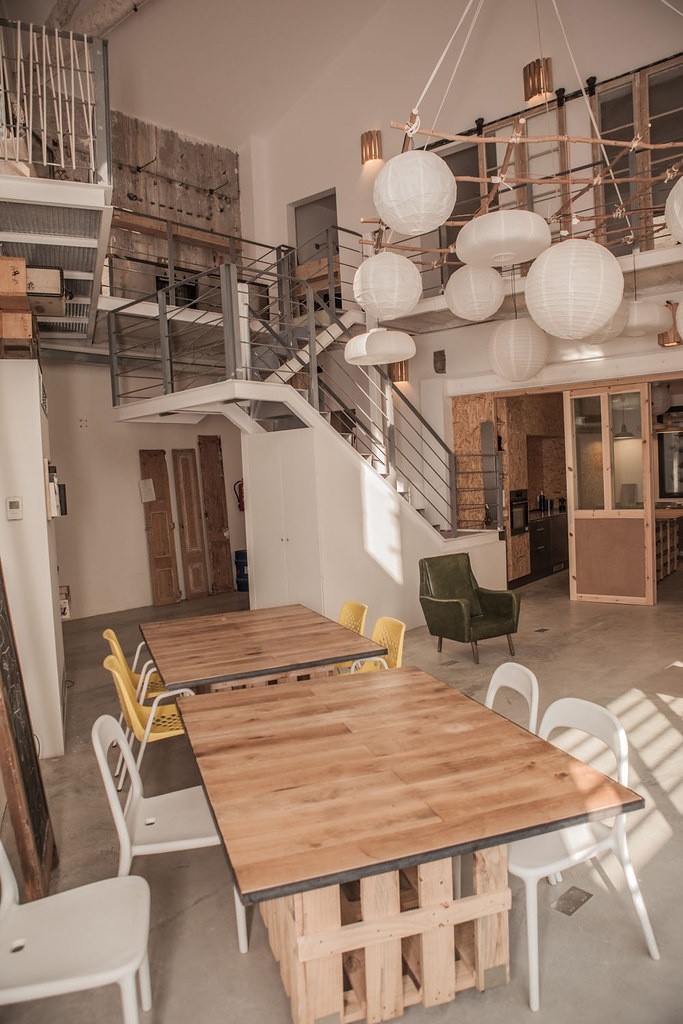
[235,551,249,590]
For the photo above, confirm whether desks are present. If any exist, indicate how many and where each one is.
[140,603,387,690]
[175,663,645,905]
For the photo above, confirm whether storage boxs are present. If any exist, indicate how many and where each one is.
[260,841,511,1024]
[202,660,355,693]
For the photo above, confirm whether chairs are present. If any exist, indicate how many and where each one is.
[418,553,522,664]
[337,599,369,635]
[485,662,539,735]
[91,715,250,954]
[508,696,660,1013]
[338,616,405,676]
[103,654,186,796]
[104,628,197,749]
[351,657,389,673]
[0,840,151,1024]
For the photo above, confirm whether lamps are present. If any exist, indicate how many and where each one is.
[523,57,554,102]
[658,299,683,348]
[388,360,410,383]
[360,129,384,169]
[612,395,635,439]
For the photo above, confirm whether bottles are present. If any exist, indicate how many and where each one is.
[536,491,567,512]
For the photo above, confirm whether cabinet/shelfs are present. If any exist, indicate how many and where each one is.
[530,513,568,572]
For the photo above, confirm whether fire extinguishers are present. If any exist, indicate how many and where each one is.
[233,478,244,511]
